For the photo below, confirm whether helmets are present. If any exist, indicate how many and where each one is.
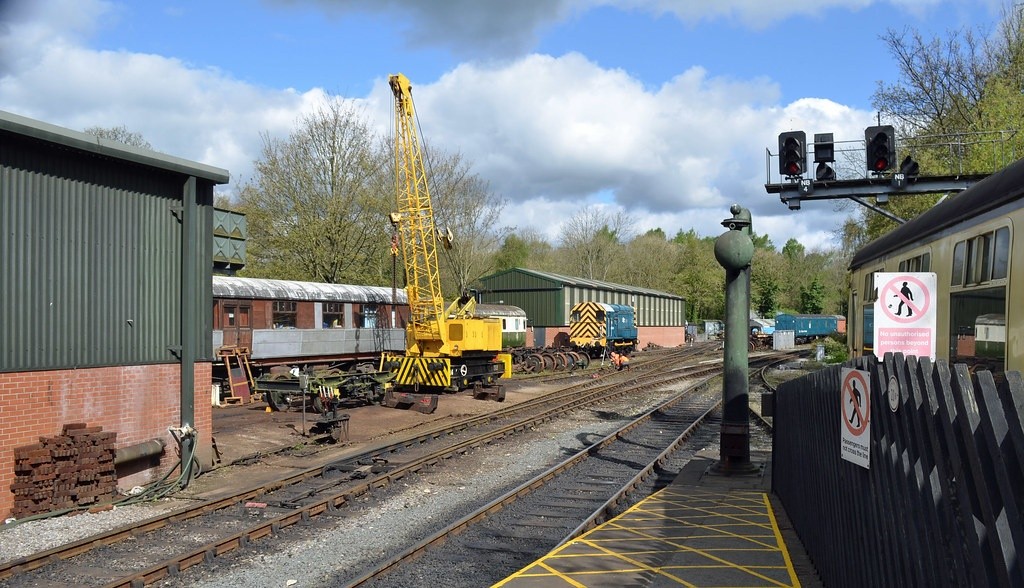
[620,353,623,355]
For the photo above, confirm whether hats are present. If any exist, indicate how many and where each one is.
[685,326,687,327]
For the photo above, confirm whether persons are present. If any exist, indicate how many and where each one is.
[609,351,630,371]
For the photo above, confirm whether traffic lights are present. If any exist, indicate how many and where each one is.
[813,133,834,161]
[864,125,897,171]
[778,130,807,174]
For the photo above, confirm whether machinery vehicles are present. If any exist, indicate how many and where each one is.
[385,71,512,394]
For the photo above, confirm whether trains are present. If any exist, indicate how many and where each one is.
[774,313,846,344]
[568,301,640,358]
